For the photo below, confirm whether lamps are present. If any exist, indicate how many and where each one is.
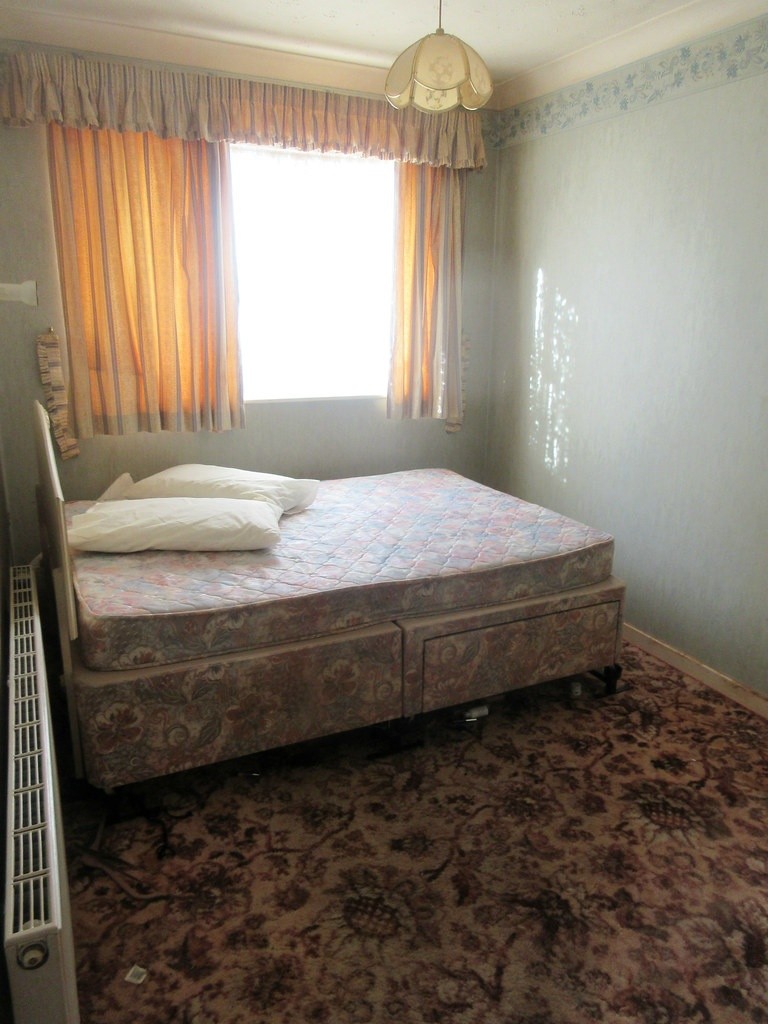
[383,0,493,115]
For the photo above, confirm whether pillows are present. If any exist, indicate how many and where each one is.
[68,496,284,556]
[115,460,320,516]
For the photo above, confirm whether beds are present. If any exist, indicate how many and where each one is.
[35,400,626,795]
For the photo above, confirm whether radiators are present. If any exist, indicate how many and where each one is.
[3,563,80,1024]
